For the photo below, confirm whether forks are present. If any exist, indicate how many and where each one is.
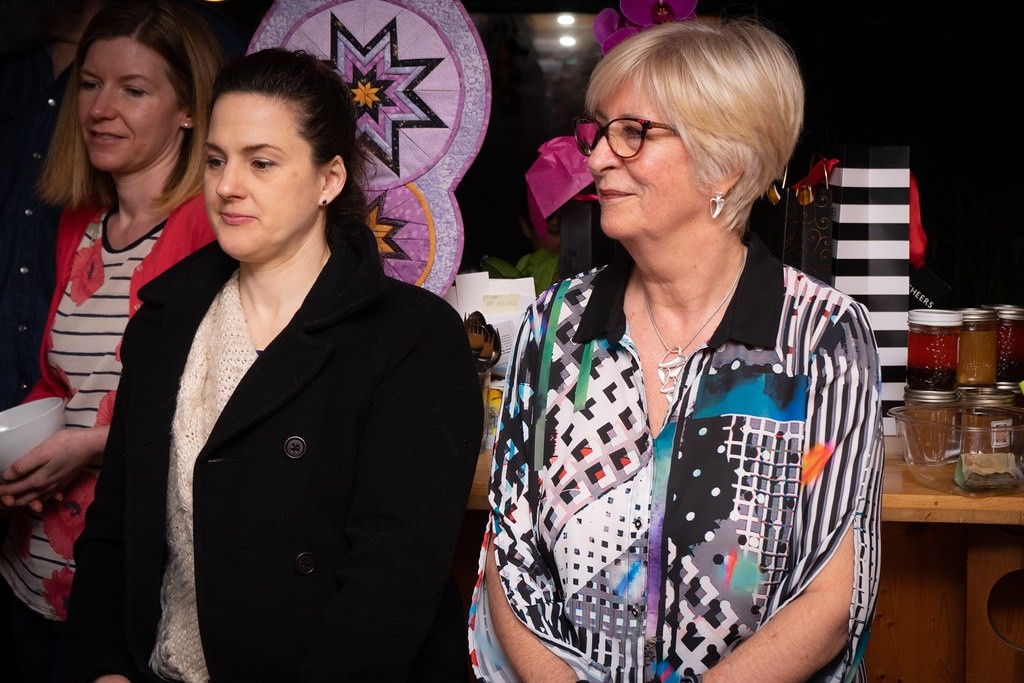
[462,310,503,392]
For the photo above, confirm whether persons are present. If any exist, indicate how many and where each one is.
[60,47,488,681]
[466,15,888,683]
[0,0,227,682]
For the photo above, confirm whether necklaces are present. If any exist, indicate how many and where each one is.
[632,245,745,406]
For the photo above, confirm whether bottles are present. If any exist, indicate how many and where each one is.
[901,382,958,464]
[906,309,960,391]
[955,307,997,386]
[958,387,1014,459]
[984,303,1024,384]
[996,381,1024,453]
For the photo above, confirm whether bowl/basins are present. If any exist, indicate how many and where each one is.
[886,401,1023,498]
[0,396,66,478]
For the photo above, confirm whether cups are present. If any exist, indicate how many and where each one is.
[475,369,494,454]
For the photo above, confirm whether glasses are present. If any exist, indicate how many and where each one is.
[571,116,680,159]
